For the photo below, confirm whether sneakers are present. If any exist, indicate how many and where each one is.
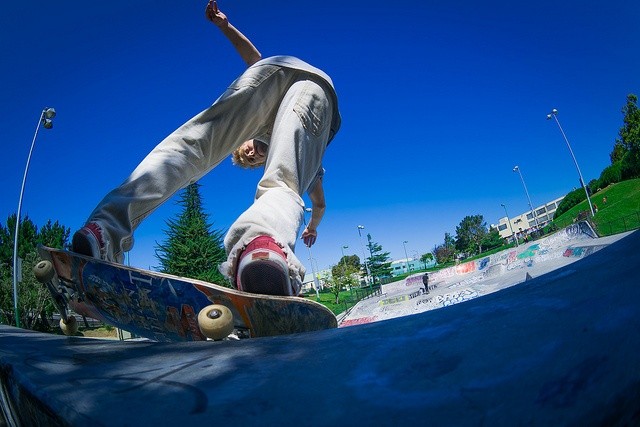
[74,221,113,260]
[235,236,295,298]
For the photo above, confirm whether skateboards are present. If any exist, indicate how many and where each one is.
[34,244,338,339]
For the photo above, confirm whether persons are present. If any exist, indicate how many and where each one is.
[422,273,429,293]
[72,1,341,299]
[529,227,538,240]
[518,228,529,243]
[602,198,607,205]
[512,231,519,247]
[537,225,545,236]
[593,204,598,213]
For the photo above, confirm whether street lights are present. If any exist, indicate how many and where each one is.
[12,107,57,326]
[340,246,352,294]
[402,240,413,272]
[501,203,517,245]
[308,257,321,294]
[512,165,539,231]
[358,224,371,278]
[546,108,594,217]
